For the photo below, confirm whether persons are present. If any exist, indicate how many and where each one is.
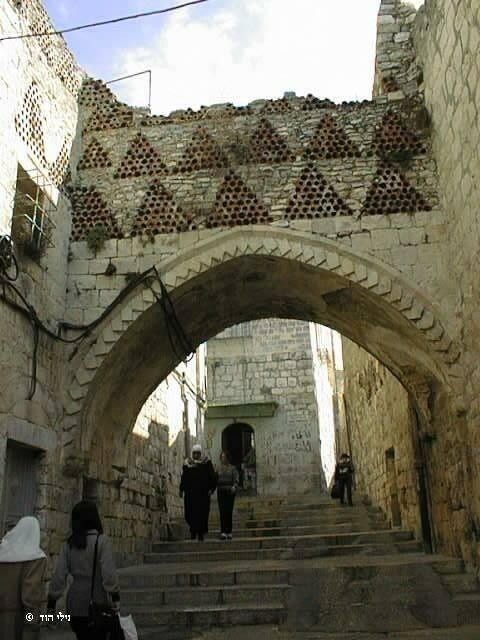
[49,499,127,640]
[212,448,239,538]
[0,515,48,640]
[334,451,356,507]
[179,443,216,539]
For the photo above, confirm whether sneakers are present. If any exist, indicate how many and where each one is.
[187,532,234,540]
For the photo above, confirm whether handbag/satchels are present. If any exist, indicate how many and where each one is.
[115,608,139,639]
[88,599,125,639]
[331,484,341,499]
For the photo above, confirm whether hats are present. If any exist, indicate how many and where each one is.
[339,454,351,460]
